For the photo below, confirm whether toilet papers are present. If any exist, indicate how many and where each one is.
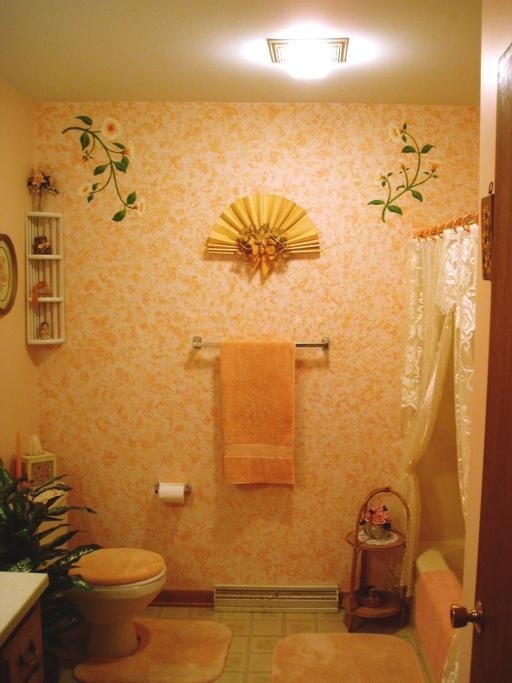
[158,484,185,505]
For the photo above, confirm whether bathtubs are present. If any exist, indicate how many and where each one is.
[414,539,466,683]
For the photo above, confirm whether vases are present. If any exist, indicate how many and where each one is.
[368,523,385,540]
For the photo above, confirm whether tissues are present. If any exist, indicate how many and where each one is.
[13,436,57,493]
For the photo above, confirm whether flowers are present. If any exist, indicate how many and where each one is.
[27,161,60,212]
[360,502,392,527]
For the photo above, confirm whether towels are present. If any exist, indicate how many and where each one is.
[218,338,297,487]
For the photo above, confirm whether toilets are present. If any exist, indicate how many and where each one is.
[28,488,167,659]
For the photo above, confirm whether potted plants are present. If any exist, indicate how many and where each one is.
[0,457,105,683]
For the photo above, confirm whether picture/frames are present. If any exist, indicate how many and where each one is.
[0,233,17,312]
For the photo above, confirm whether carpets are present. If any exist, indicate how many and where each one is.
[74,618,234,683]
[271,630,427,682]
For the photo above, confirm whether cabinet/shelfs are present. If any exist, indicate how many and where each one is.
[25,212,65,346]
[0,593,47,683]
[342,487,412,634]
